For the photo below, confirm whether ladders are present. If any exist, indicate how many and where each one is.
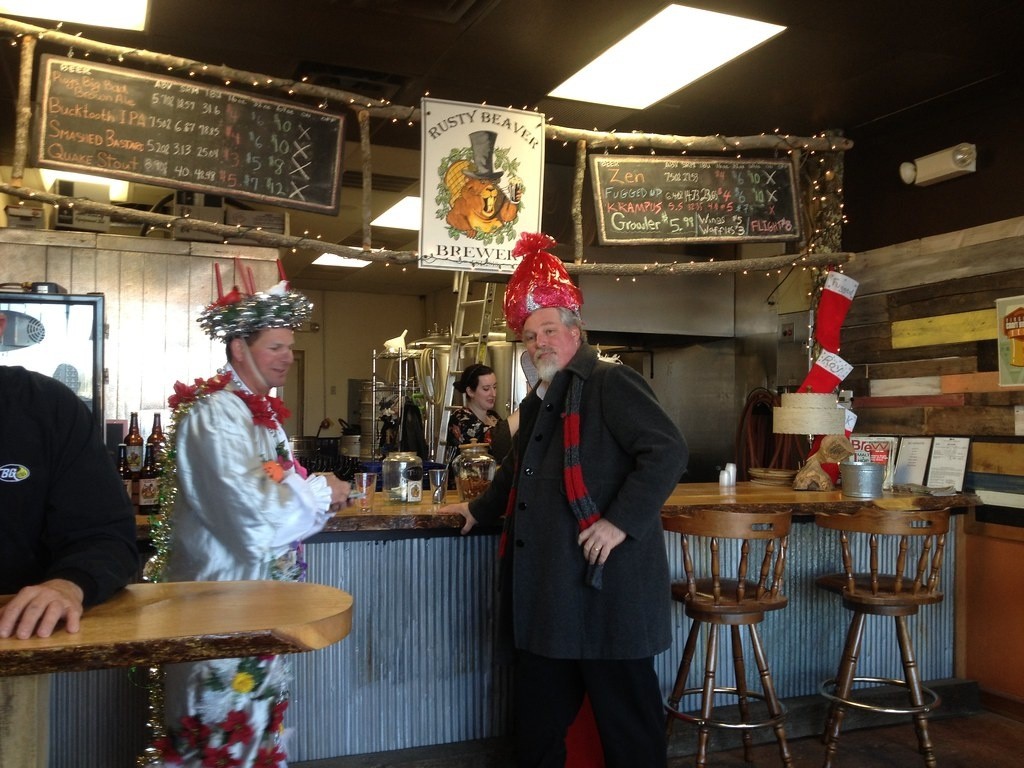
[435,270,497,465]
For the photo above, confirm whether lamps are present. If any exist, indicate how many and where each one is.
[899,142,979,189]
[0,0,153,35]
[293,322,320,333]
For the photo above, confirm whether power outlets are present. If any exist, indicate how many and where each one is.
[321,418,330,429]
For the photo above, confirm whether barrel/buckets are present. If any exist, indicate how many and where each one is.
[838,461,886,498]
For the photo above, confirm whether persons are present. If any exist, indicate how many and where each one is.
[447,365,502,491]
[156,281,350,768]
[436,233,690,768]
[0,366,137,768]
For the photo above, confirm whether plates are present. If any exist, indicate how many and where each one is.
[747,467,798,486]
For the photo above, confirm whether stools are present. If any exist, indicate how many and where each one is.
[813,504,959,768]
[661,510,797,768]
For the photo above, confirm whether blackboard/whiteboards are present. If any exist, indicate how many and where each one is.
[27,53,347,216]
[588,154,802,246]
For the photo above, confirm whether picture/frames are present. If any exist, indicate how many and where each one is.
[925,436,972,491]
[895,436,934,486]
[417,99,546,274]
[866,434,901,490]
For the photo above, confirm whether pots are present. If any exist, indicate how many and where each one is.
[288,435,360,460]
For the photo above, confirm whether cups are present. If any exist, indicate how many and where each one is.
[353,473,377,513]
[719,462,737,488]
[427,469,449,506]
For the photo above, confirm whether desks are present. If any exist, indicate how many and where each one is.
[0,581,355,768]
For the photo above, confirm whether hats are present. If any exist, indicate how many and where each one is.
[503,232,585,340]
[196,258,314,344]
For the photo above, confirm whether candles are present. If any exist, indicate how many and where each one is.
[277,258,291,291]
[248,267,256,293]
[215,262,223,299]
[237,258,253,294]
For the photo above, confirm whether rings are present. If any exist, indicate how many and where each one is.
[594,547,599,551]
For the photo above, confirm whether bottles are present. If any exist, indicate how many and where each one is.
[137,442,160,515]
[146,412,166,451]
[451,447,497,502]
[124,411,144,481]
[115,444,132,503]
[382,451,423,506]
